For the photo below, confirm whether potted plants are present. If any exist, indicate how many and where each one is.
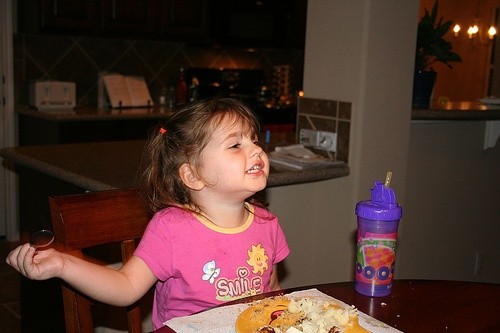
[412,0,464,107]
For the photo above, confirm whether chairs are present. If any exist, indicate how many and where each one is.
[48,186,164,333]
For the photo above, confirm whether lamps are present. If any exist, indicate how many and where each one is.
[453,11,497,50]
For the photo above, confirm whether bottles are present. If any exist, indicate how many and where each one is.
[355,182,403,296]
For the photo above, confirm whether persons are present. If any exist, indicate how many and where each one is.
[6,98,290,331]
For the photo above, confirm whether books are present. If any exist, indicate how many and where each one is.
[267,144,320,170]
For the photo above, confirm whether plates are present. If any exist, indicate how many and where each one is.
[235,295,372,333]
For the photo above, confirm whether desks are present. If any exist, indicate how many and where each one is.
[148,279,500,333]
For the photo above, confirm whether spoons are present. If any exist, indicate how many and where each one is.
[29,230,54,250]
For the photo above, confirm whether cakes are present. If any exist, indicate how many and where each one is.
[235,294,374,333]
